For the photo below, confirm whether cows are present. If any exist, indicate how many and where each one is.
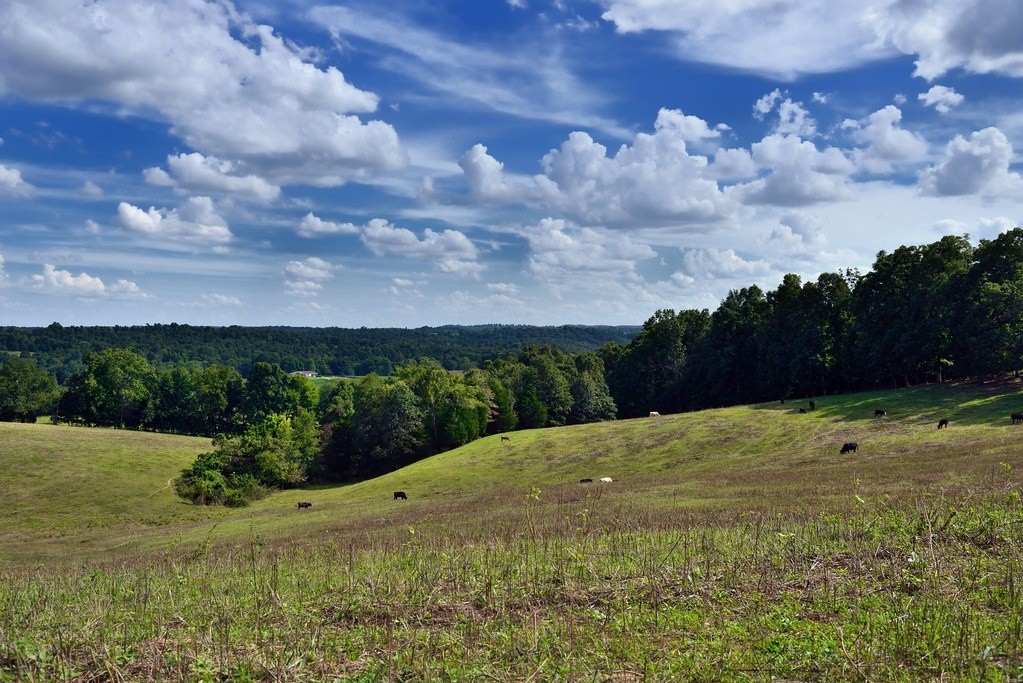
[649,411,660,416]
[1011,412,1023,424]
[600,477,612,483]
[808,400,815,410]
[297,502,312,510]
[781,398,784,404]
[799,407,806,413]
[936,418,948,429]
[840,442,859,454]
[874,409,887,418]
[393,491,408,500]
[501,436,510,442]
[579,478,593,483]
